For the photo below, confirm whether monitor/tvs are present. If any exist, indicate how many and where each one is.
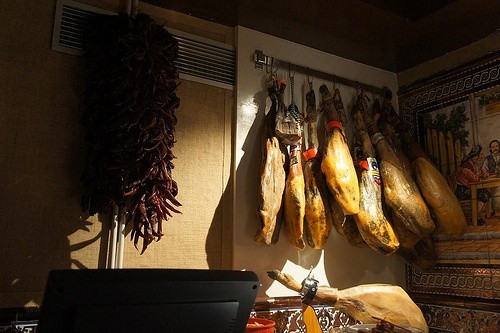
[36,270,259,333]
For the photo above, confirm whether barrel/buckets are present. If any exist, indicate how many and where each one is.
[246,317,276,333]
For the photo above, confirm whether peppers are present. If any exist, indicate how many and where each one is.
[74,13,183,255]
